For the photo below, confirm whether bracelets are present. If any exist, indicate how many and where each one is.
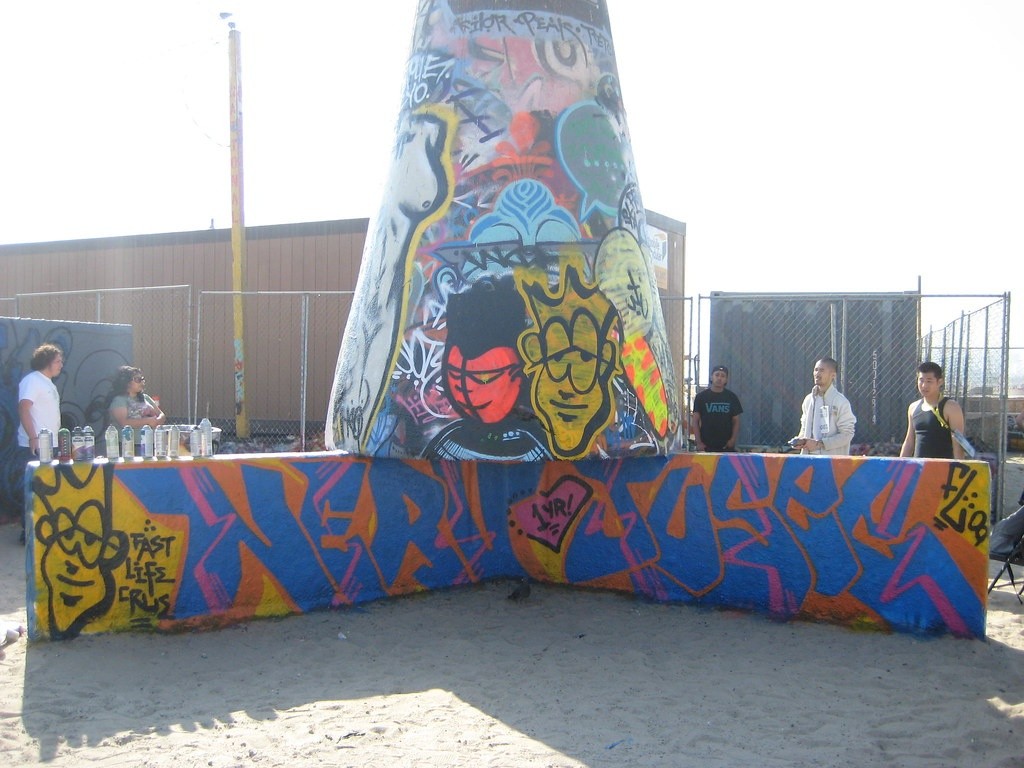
[29,437,38,441]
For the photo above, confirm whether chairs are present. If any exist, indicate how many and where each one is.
[988,530,1024,600]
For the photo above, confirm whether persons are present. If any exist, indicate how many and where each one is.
[693,365,743,452]
[900,362,965,459]
[788,357,857,454]
[17,344,64,544]
[104,366,165,456]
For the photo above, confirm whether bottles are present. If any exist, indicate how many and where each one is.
[38,428,51,462]
[105,424,119,458]
[200,418,212,457]
[58,427,70,460]
[168,423,180,456]
[140,425,153,458]
[122,425,134,457]
[154,425,166,456]
[48,431,53,458]
[83,426,95,459]
[72,425,83,460]
[190,425,200,457]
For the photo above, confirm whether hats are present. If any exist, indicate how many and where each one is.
[711,365,728,386]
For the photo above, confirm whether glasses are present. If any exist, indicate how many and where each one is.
[128,374,147,384]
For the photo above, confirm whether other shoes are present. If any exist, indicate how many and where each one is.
[19,529,25,544]
